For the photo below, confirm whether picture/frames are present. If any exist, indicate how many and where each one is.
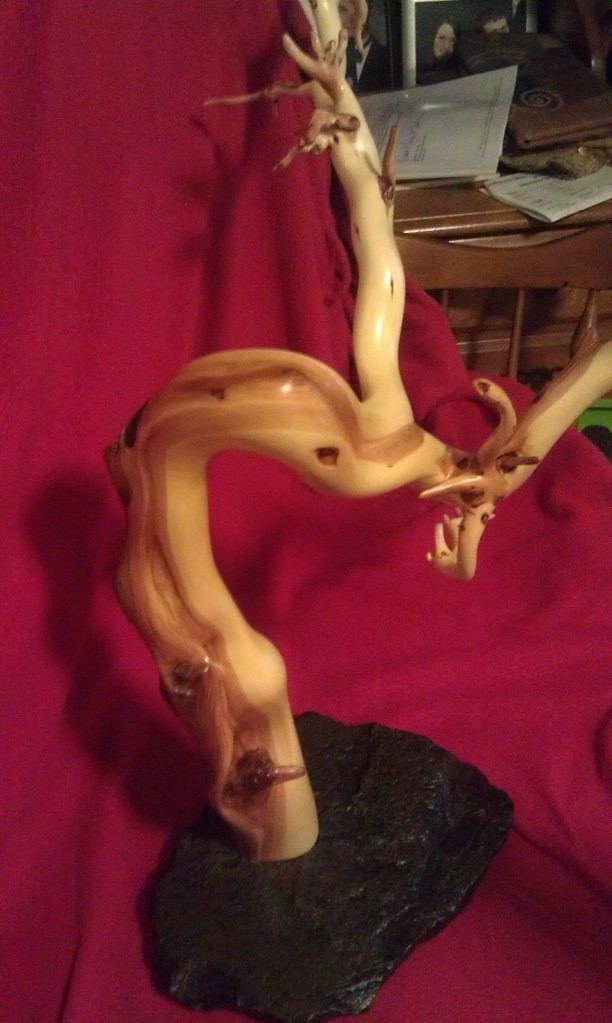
[383,0,535,91]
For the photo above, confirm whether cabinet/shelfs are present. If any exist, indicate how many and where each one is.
[390,176,612,372]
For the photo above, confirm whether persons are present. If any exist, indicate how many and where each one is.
[427,15,461,66]
[339,0,391,93]
[475,8,511,36]
